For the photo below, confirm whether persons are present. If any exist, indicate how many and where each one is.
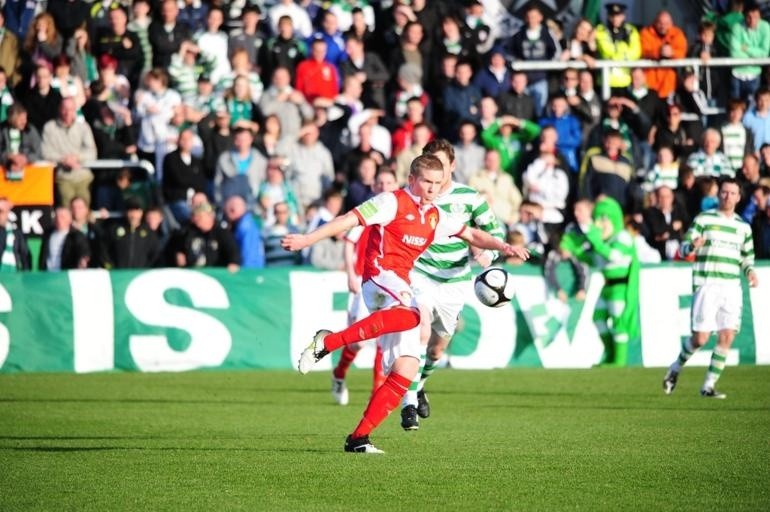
[279,153,533,457]
[328,167,399,406]
[1,0,770,273]
[390,139,505,433]
[661,177,759,399]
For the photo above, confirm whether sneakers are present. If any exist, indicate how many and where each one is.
[400,404,420,431]
[298,329,334,376]
[593,359,626,371]
[345,433,386,455]
[700,386,727,400]
[662,363,681,395]
[331,374,350,405]
[415,390,431,418]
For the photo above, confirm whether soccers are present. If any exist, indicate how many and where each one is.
[474,267,516,308]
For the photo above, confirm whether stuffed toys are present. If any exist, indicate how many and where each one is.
[560,193,637,369]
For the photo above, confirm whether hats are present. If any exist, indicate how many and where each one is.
[604,3,627,16]
